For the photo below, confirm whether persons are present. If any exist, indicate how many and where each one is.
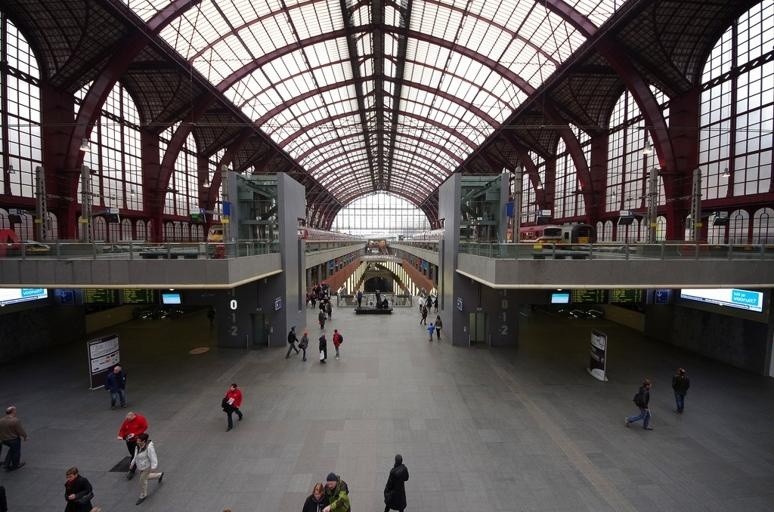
[623,376,655,431]
[383,452,408,511]
[207,306,215,327]
[281,282,446,363]
[222,382,243,430]
[0,402,28,468]
[303,481,325,512]
[2,486,9,512]
[668,367,692,416]
[62,467,101,511]
[130,433,165,505]
[116,411,148,479]
[105,364,129,409]
[324,473,352,512]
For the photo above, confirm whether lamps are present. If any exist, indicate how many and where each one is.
[79,136,91,153]
[722,168,731,178]
[536,179,543,189]
[200,175,212,187]
[642,140,653,155]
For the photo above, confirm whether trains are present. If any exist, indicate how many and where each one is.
[295,228,355,239]
[508,224,563,248]
[562,223,598,256]
[207,226,225,258]
[411,227,440,241]
